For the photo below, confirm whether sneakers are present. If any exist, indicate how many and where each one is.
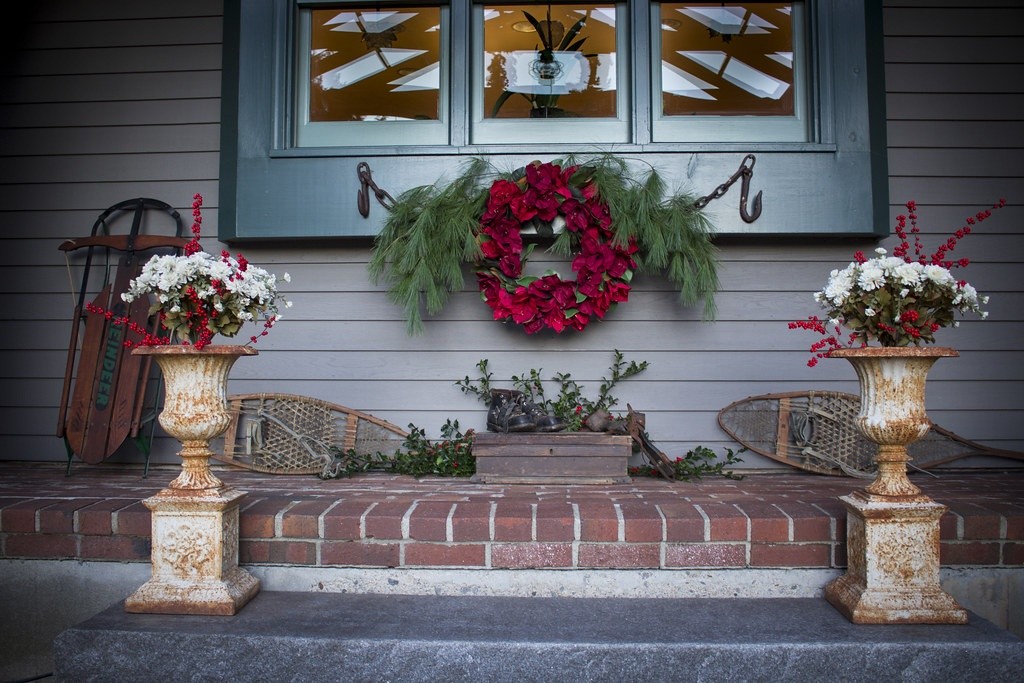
[486,388,535,435]
[514,390,565,432]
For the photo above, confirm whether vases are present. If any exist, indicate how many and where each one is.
[134,343,256,493]
[833,341,959,503]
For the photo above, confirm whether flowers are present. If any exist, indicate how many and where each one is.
[85,196,295,351]
[316,356,748,479]
[789,195,1008,367]
[476,158,649,333]
